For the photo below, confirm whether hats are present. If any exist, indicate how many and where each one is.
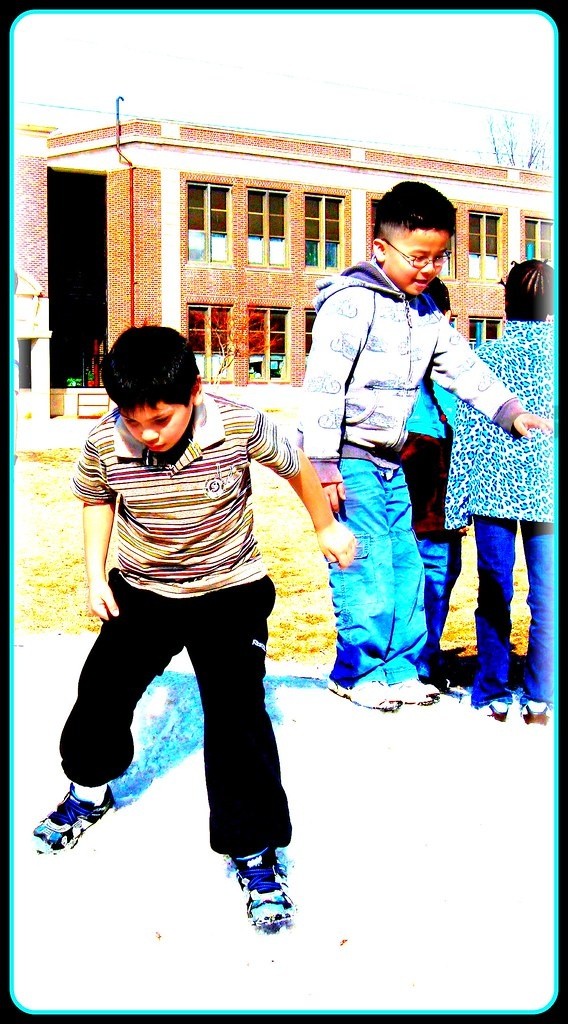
[502,259,554,323]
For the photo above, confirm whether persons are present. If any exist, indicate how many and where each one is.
[414,276,466,694]
[304,179,554,726]
[32,325,359,934]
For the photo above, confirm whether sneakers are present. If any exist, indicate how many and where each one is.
[391,677,439,706]
[220,846,294,934]
[328,676,402,712]
[32,784,116,856]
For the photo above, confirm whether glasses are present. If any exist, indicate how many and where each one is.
[383,237,455,271]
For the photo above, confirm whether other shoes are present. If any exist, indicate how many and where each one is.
[478,701,508,721]
[521,701,551,726]
